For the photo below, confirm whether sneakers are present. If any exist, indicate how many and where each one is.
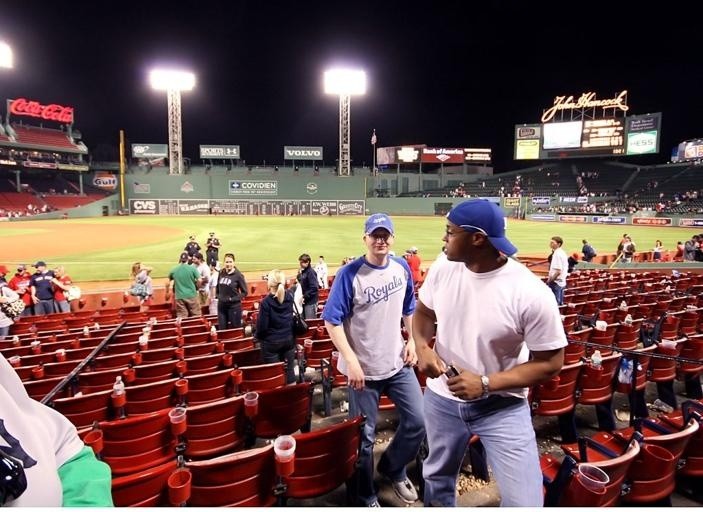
[360,495,380,507]
[377,464,418,503]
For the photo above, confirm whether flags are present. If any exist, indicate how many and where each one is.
[370,135,376,144]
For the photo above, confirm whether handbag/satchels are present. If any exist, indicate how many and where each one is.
[131,284,149,296]
[65,285,81,301]
[1,300,26,318]
[293,316,309,335]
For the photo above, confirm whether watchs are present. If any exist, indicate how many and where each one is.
[479,374,489,397]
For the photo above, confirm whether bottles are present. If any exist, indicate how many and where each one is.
[624,314,633,326]
[620,301,627,310]
[113,376,124,395]
[211,325,217,336]
[12,335,19,343]
[94,323,100,329]
[590,350,603,369]
[84,326,89,335]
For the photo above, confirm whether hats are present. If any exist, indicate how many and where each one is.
[181,233,216,266]
[365,215,394,238]
[0,262,46,275]
[446,200,517,256]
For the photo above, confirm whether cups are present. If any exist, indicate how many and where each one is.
[596,320,677,349]
[575,269,636,282]
[578,462,611,492]
[139,317,155,345]
[274,435,296,461]
[168,407,187,422]
[243,391,259,405]
[9,341,65,363]
[304,339,339,360]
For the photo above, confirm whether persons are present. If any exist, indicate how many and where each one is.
[131,261,154,314]
[0,153,81,165]
[0,353,115,508]
[165,231,248,330]
[674,233,702,263]
[314,256,329,289]
[651,240,663,262]
[548,243,555,264]
[411,196,568,507]
[617,234,636,263]
[0,188,87,221]
[342,245,429,298]
[547,236,569,305]
[567,253,578,277]
[295,253,319,319]
[0,260,82,336]
[581,239,597,262]
[324,213,427,507]
[251,269,297,389]
[441,170,703,216]
